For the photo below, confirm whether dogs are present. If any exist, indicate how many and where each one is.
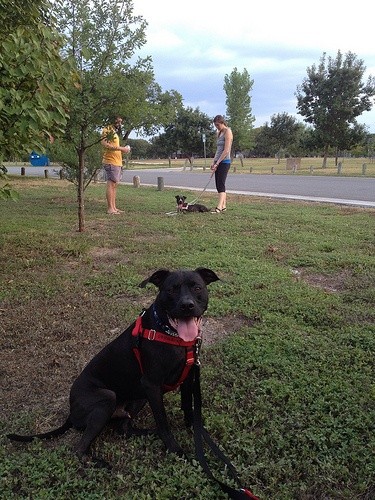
[5,268,222,461]
[165,194,213,216]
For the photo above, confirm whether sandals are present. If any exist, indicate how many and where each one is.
[209,206,226,214]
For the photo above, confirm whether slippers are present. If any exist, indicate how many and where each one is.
[106,209,126,215]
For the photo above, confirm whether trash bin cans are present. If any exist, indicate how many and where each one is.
[29,148,46,166]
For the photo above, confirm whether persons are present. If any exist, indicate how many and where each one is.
[210,115,233,213]
[101,116,128,214]
